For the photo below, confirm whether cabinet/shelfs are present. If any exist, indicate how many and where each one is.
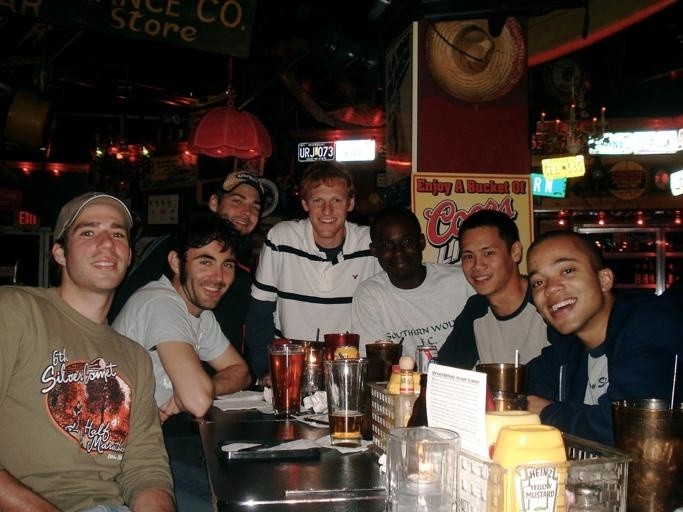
[531,196,683,297]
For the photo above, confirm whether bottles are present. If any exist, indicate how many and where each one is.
[629,257,683,284]
[388,357,432,429]
[298,342,325,397]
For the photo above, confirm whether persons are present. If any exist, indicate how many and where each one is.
[102,166,280,356]
[351,206,479,373]
[520,230,683,445]
[1,192,178,512]
[245,159,373,391]
[108,208,253,511]
[434,208,554,370]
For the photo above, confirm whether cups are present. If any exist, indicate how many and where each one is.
[267,341,306,416]
[324,334,361,359]
[323,361,367,443]
[388,426,459,512]
[366,342,403,383]
[476,362,529,410]
[615,397,683,510]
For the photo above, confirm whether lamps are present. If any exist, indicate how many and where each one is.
[189,53,274,161]
[90,116,157,186]
[531,70,609,155]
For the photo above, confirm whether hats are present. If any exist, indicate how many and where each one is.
[52,192,135,242]
[219,169,279,218]
[427,16,527,103]
